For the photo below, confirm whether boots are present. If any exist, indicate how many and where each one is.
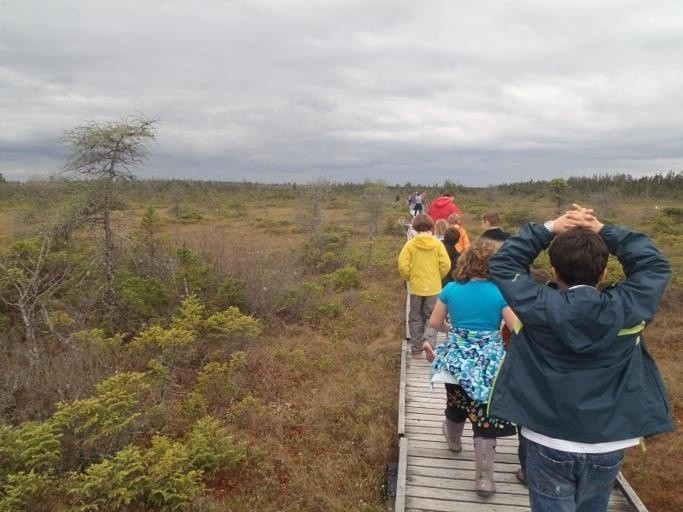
[473,437,497,496]
[442,416,464,451]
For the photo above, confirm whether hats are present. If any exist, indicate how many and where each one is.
[481,227,511,240]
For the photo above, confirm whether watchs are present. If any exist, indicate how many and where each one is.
[544,221,554,232]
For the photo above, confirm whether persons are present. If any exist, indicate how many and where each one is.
[433,212,470,289]
[501,269,549,483]
[430,191,459,221]
[397,213,452,363]
[479,212,511,243]
[424,239,516,495]
[408,191,426,215]
[486,203,676,512]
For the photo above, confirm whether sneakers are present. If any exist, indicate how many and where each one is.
[412,342,436,364]
[517,469,528,486]
